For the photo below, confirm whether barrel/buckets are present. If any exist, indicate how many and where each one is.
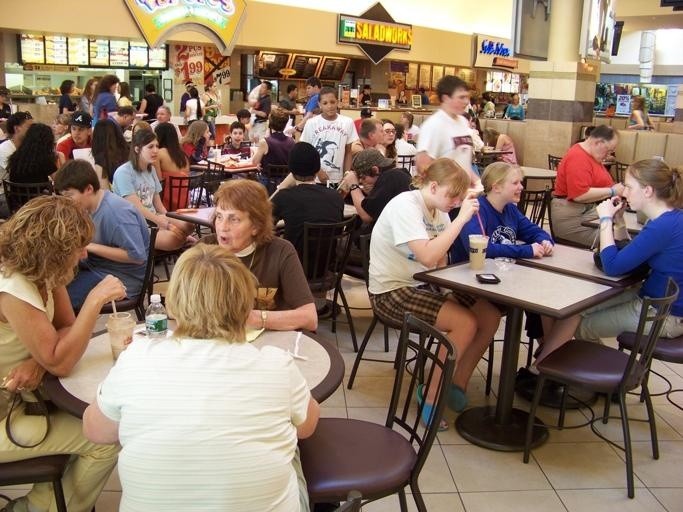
[446,381,468,413]
[417,384,449,431]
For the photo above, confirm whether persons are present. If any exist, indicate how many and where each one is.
[574,125,616,173]
[549,125,632,251]
[416,75,516,209]
[369,157,502,430]
[2,74,417,313]
[624,95,655,132]
[450,161,582,396]
[606,104,617,117]
[2,196,124,511]
[83,241,320,512]
[512,158,683,393]
[201,179,318,330]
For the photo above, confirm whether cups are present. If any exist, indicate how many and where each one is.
[383,130,397,136]
[23,111,33,120]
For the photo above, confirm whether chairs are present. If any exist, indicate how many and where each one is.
[0,136,683,512]
[515,342,567,393]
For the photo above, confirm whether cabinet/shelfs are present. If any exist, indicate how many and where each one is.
[110,314,135,358]
[208,146,215,170]
[147,292,165,343]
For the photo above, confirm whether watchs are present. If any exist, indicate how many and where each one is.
[260,309,268,329]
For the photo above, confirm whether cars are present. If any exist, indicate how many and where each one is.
[468,234,486,270]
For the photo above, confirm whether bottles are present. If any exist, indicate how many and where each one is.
[364,85,370,89]
[360,108,374,117]
[71,111,93,127]
[288,142,320,176]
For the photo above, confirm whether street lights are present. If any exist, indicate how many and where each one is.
[21,378,58,415]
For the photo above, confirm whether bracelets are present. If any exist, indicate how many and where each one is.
[495,258,516,271]
